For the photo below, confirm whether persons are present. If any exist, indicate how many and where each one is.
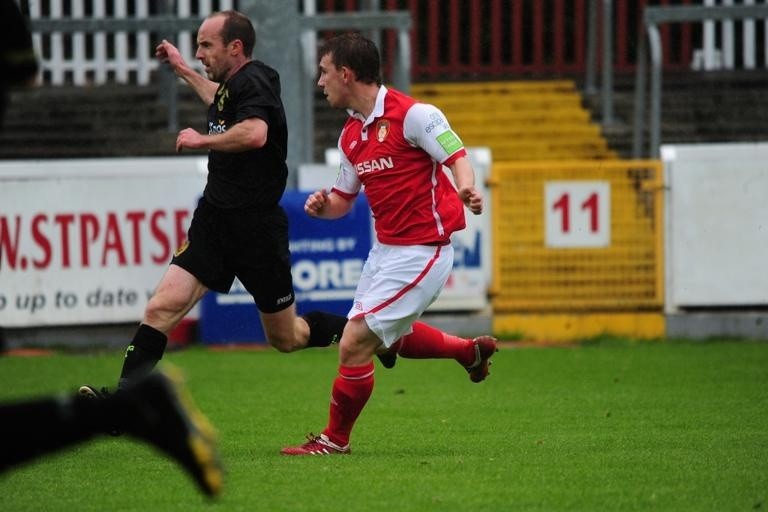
[79,10,398,401]
[0,0,40,93]
[0,363,225,496]
[280,31,500,457]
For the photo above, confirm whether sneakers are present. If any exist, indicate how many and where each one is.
[79,385,124,436]
[377,351,396,368]
[464,336,498,382]
[280,433,350,455]
[134,373,221,494]
